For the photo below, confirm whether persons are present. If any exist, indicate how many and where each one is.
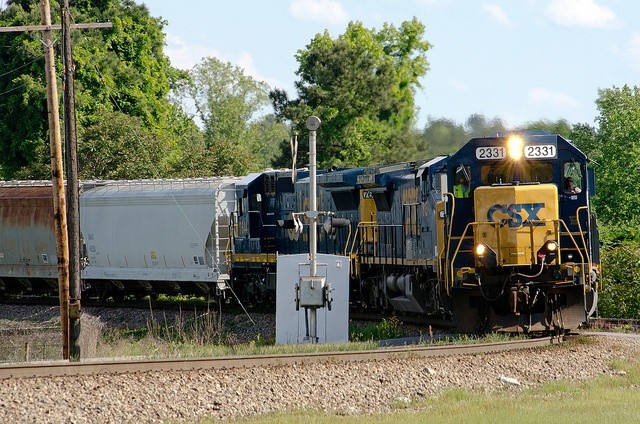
[454,175,470,198]
[566,176,574,192]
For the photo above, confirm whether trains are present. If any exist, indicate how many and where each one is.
[0,131,603,333]
